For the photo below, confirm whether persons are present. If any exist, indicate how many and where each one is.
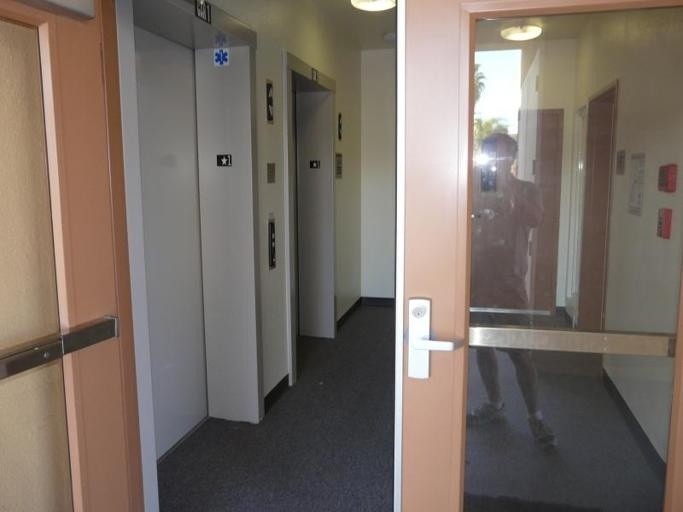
[465,134,560,450]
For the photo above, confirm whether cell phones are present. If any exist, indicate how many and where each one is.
[480,138,497,192]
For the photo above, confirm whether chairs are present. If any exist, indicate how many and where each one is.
[528,412,557,450]
[467,400,505,428]
